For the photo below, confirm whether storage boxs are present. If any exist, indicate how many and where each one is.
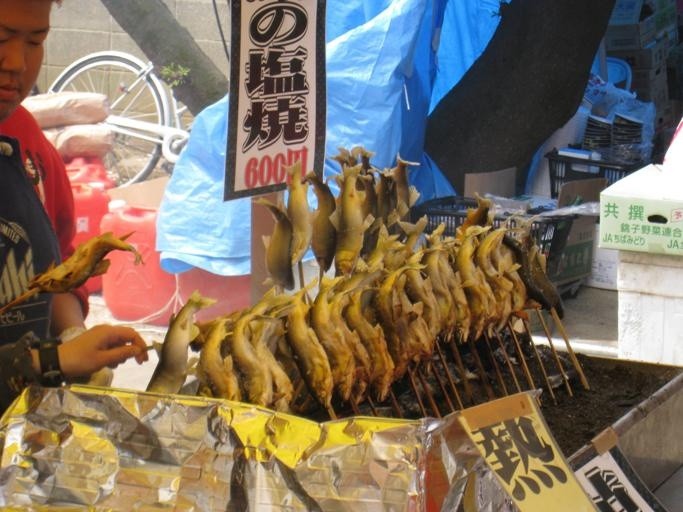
[458,166,608,282]
[596,0,681,262]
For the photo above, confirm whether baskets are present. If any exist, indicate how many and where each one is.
[407,196,554,268]
[544,142,639,201]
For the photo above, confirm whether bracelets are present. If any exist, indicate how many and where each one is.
[29,334,62,388]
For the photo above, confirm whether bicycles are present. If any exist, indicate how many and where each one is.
[45,50,193,186]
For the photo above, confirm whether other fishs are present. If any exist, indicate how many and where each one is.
[27,231,143,296]
[141,146,566,415]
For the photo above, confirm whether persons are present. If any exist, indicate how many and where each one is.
[0,135,66,432]
[0,2,117,402]
[2,325,150,409]
